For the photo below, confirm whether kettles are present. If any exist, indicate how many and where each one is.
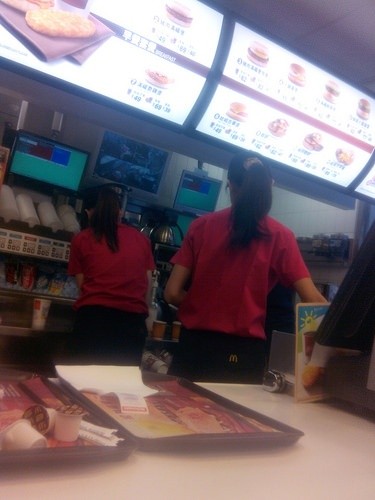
[150,219,184,247]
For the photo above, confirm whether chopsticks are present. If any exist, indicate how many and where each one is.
[41,375,72,406]
[17,381,50,408]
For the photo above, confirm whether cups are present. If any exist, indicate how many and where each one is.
[172,321,181,340]
[22,261,38,289]
[142,346,173,376]
[55,0,94,16]
[0,183,81,234]
[153,320,167,340]
[31,298,53,329]
[304,332,317,362]
[0,419,48,448]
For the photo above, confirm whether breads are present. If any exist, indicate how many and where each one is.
[1,0,95,38]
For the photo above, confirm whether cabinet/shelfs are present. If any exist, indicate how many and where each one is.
[0,216,78,337]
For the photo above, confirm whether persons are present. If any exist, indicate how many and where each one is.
[67,187,155,356]
[302,219,375,396]
[164,150,329,380]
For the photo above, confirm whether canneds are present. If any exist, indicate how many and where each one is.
[295,233,350,262]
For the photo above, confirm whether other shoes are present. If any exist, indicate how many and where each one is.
[300,342,336,391]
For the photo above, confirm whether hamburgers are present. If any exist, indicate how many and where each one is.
[355,99,371,120]
[289,63,305,86]
[166,1,194,27]
[323,81,341,104]
[145,71,169,88]
[247,47,268,65]
[227,102,248,122]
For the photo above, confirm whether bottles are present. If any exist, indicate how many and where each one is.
[296,232,350,256]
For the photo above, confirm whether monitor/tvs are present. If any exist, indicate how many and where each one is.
[7,130,91,195]
[90,128,170,196]
[174,169,223,214]
